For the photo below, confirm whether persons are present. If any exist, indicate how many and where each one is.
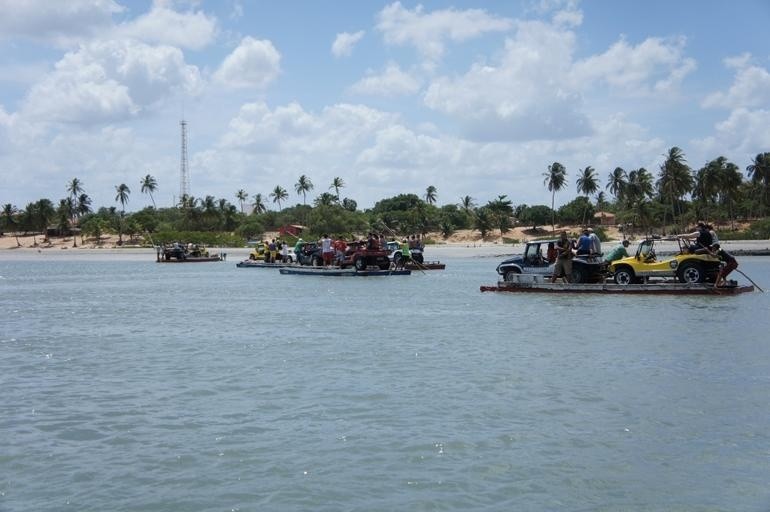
[152,240,199,261]
[544,227,630,284]
[677,219,738,287]
[264,232,424,269]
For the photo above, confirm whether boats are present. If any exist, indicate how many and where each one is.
[152,254,450,278]
[476,280,757,296]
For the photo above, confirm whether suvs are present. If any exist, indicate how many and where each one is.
[246,237,406,270]
[606,232,727,285]
[495,234,619,285]
